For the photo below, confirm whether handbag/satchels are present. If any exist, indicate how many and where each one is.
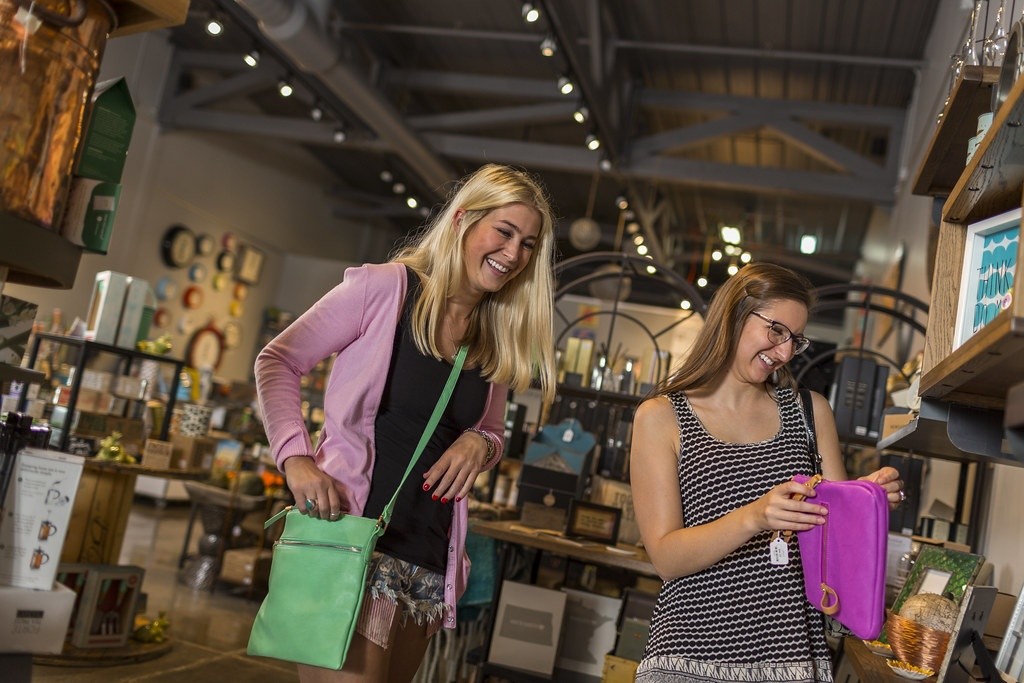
[247,504,378,671]
[791,474,888,642]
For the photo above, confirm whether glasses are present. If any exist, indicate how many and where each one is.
[750,311,810,356]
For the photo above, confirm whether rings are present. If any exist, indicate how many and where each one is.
[305,498,316,510]
[331,511,340,516]
[899,489,904,501]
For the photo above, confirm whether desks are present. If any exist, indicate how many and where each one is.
[181,480,289,597]
[466,514,662,683]
[834,636,933,683]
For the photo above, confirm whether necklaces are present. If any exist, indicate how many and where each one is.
[446,307,459,360]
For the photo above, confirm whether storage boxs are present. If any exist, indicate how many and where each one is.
[486,564,662,683]
[0,371,277,654]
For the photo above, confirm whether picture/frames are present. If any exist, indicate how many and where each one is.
[950,208,1021,353]
[563,499,623,546]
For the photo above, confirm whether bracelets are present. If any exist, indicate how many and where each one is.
[464,427,494,463]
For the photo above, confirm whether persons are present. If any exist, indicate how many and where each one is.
[630,262,905,682]
[254,163,556,683]
